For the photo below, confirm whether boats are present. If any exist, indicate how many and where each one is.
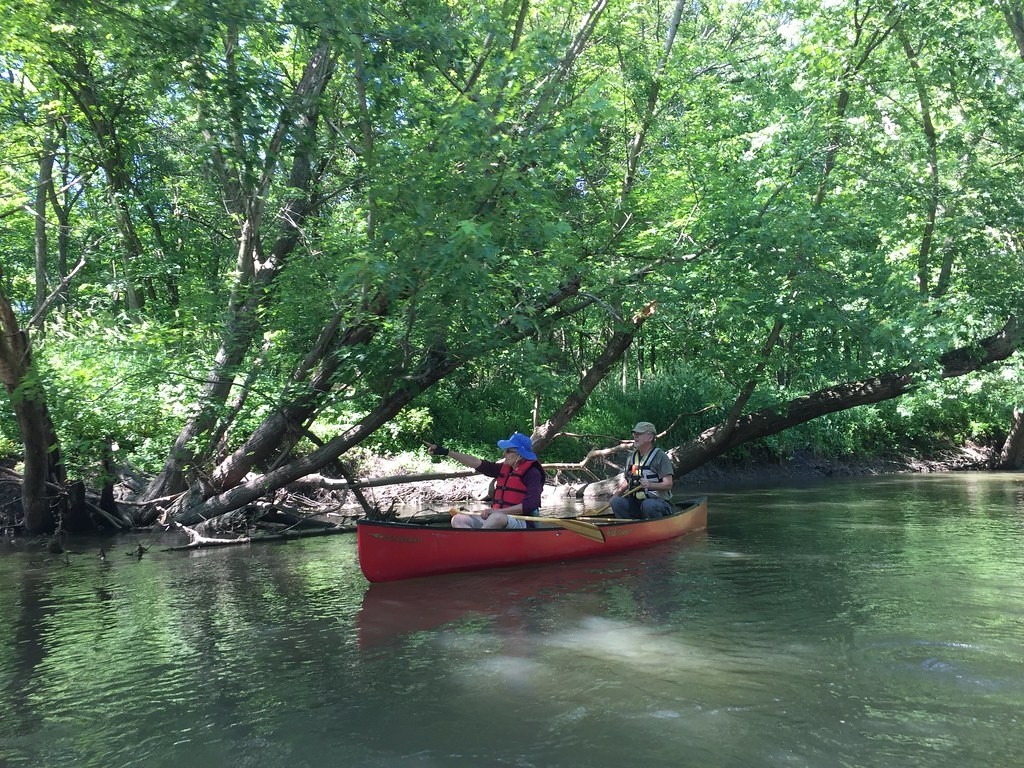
[356,494,710,584]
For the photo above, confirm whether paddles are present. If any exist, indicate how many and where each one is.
[576,517,649,523]
[448,507,607,544]
[577,483,643,516]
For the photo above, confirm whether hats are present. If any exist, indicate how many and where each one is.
[631,422,657,436]
[497,431,537,460]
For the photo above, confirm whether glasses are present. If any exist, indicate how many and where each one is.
[506,449,516,454]
[632,433,643,437]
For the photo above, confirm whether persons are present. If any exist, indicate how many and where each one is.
[422,433,545,530]
[608,422,674,521]
